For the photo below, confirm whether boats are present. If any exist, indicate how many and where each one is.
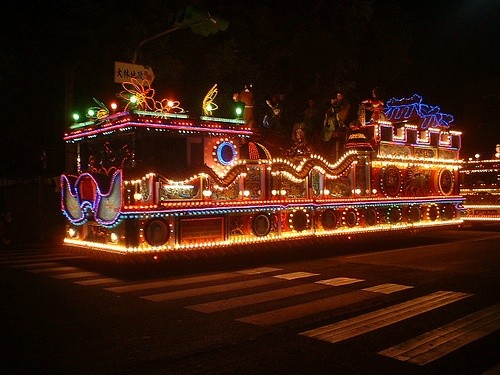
[57,61,467,274]
[456,144,500,223]
